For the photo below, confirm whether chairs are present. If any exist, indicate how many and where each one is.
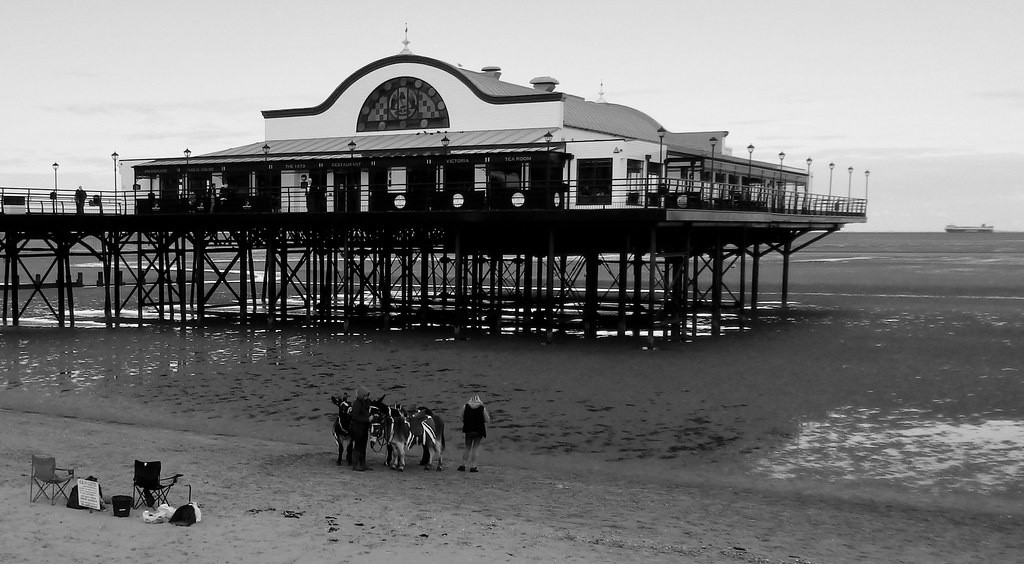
[30,455,74,505]
[132,460,183,512]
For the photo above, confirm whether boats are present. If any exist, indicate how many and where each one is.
[945,224,993,233]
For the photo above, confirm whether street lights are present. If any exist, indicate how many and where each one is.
[348,140,356,212]
[865,170,870,214]
[184,148,191,198]
[111,151,119,214]
[440,136,450,209]
[746,144,755,209]
[709,137,718,208]
[846,166,853,212]
[52,162,59,213]
[544,131,553,210]
[778,152,786,212]
[805,158,813,212]
[657,126,666,208]
[262,144,270,213]
[828,162,834,212]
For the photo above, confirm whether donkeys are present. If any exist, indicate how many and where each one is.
[331,392,355,467]
[366,392,446,472]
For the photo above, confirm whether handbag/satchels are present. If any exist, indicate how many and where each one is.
[67,476,103,510]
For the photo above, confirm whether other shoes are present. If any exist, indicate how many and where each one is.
[470,468,478,472]
[458,466,465,471]
[353,465,365,470]
[362,464,368,469]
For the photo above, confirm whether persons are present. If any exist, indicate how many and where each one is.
[305,178,313,213]
[76,186,87,214]
[208,183,216,214]
[457,394,490,472]
[350,387,372,471]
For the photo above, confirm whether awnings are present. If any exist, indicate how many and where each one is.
[133,128,559,177]
